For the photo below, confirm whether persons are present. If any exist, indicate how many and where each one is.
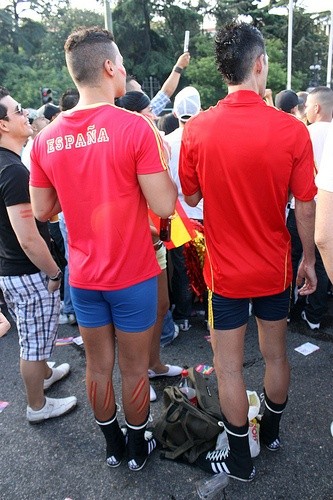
[0,52,333,399]
[0,86,77,425]
[29,27,178,472]
[178,22,317,482]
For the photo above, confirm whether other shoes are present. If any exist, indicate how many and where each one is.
[25,396,78,422]
[43,363,70,391]
[173,324,179,339]
[302,311,323,330]
[149,364,184,378]
[58,313,75,324]
[149,384,158,401]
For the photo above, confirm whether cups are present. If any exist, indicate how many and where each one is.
[194,472,229,500]
[158,131,165,141]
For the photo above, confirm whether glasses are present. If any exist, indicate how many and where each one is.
[3,103,23,121]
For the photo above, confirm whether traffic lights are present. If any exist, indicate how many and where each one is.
[40,87,54,106]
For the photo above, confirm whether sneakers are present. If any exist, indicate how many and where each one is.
[106,427,127,467]
[198,449,258,482]
[254,419,284,451]
[125,427,158,470]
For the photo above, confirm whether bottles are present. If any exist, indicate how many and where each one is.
[178,370,197,409]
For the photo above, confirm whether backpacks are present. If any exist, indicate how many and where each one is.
[153,367,226,462]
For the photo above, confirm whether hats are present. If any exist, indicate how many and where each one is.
[274,88,304,112]
[26,107,44,124]
[42,102,62,119]
[174,85,201,122]
